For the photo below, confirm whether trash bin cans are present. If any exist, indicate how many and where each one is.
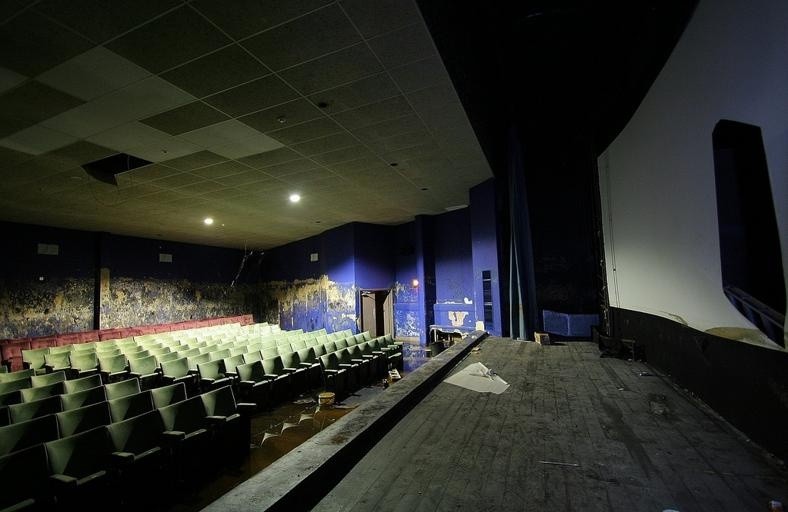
[318,392,335,406]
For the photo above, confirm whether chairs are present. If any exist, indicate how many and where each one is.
[2,369,259,510]
[1,314,403,414]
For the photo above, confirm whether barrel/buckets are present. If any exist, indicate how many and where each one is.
[429,341,444,357]
[318,392,336,406]
